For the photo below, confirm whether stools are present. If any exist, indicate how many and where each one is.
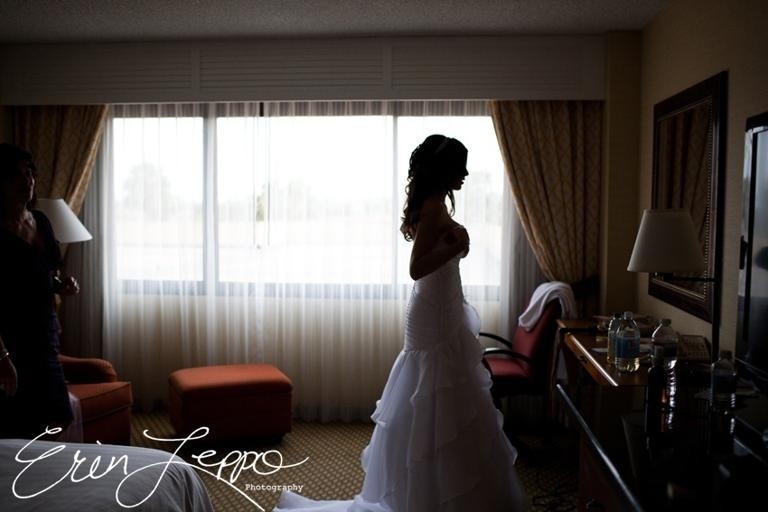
[169,364,293,455]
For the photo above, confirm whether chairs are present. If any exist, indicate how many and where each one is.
[478,281,570,467]
[58,351,133,442]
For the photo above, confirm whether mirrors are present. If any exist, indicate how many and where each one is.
[644,69,730,328]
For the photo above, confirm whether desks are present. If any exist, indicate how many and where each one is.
[551,316,683,389]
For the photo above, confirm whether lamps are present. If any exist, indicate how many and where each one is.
[37,198,93,244]
[625,205,719,288]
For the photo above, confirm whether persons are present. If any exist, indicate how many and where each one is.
[273,134,526,512]
[0,149,80,439]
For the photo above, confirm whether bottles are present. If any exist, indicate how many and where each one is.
[606,311,678,397]
[710,351,741,410]
